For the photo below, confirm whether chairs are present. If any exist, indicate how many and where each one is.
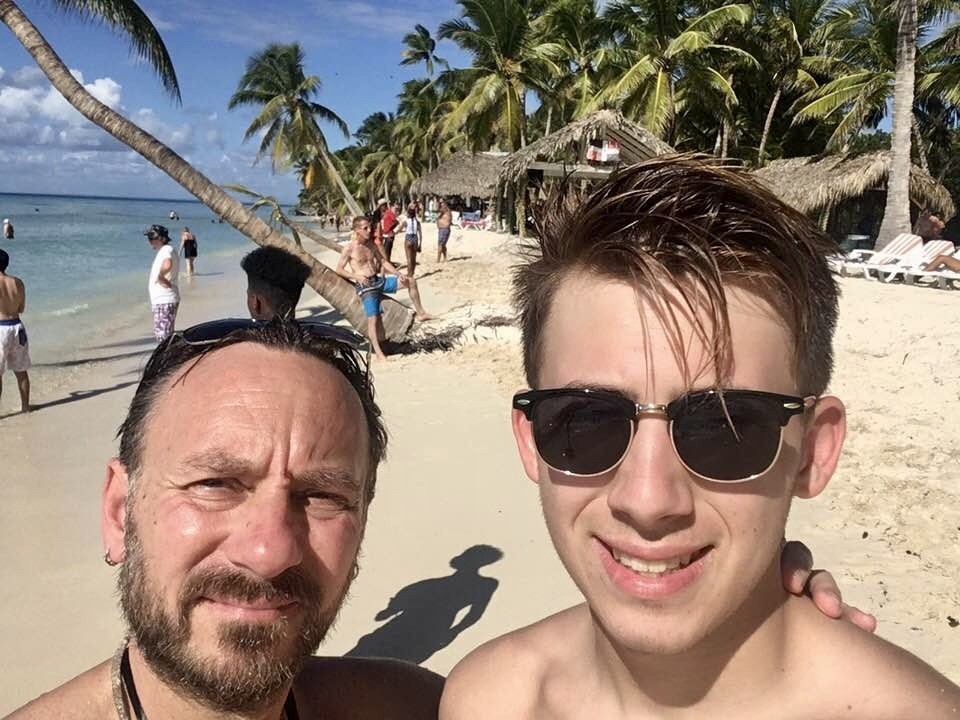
[825,234,960,290]
[451,211,507,231]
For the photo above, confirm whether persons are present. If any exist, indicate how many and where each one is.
[335,216,438,361]
[178,227,198,276]
[144,224,179,343]
[170,210,177,220]
[3,218,15,239]
[0,247,40,412]
[438,150,960,720]
[373,198,452,277]
[240,246,311,320]
[5,315,876,720]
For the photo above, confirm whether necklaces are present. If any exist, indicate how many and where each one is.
[111,634,299,720]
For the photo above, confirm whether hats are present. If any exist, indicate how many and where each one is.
[3,218,9,223]
[143,224,172,244]
[375,198,391,210]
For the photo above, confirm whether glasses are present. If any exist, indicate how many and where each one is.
[362,224,373,232]
[513,386,819,482]
[164,315,373,407]
